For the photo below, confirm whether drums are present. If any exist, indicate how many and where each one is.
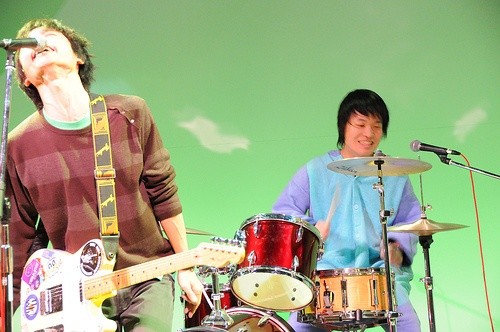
[200,307,296,332]
[294,268,399,331]
[230,214,322,311]
[184,283,237,329]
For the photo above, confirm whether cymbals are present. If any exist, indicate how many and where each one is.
[386,220,469,236]
[160,226,212,235]
[327,157,433,177]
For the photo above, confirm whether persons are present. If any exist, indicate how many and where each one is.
[273,89,425,332]
[0,17,205,332]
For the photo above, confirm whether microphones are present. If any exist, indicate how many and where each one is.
[0,32,48,52]
[410,140,460,155]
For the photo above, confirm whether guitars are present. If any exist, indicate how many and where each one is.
[19,238,246,331]
